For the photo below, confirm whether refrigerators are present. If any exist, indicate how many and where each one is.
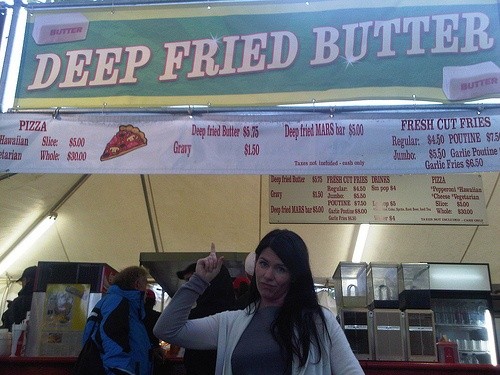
[428,263,499,366]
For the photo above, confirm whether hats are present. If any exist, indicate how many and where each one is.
[176,262,197,280]
[16,266,37,282]
[146,289,155,299]
[245,251,256,276]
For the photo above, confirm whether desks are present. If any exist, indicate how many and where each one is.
[0,357,77,375]
[358,360,500,375]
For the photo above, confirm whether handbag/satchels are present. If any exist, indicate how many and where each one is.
[72,337,106,375]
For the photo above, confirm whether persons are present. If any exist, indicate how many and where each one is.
[153,228,365,375]
[142,250,256,375]
[73,266,154,375]
[0,265,38,332]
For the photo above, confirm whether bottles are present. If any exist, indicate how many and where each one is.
[15,330,25,356]
[433,304,485,325]
